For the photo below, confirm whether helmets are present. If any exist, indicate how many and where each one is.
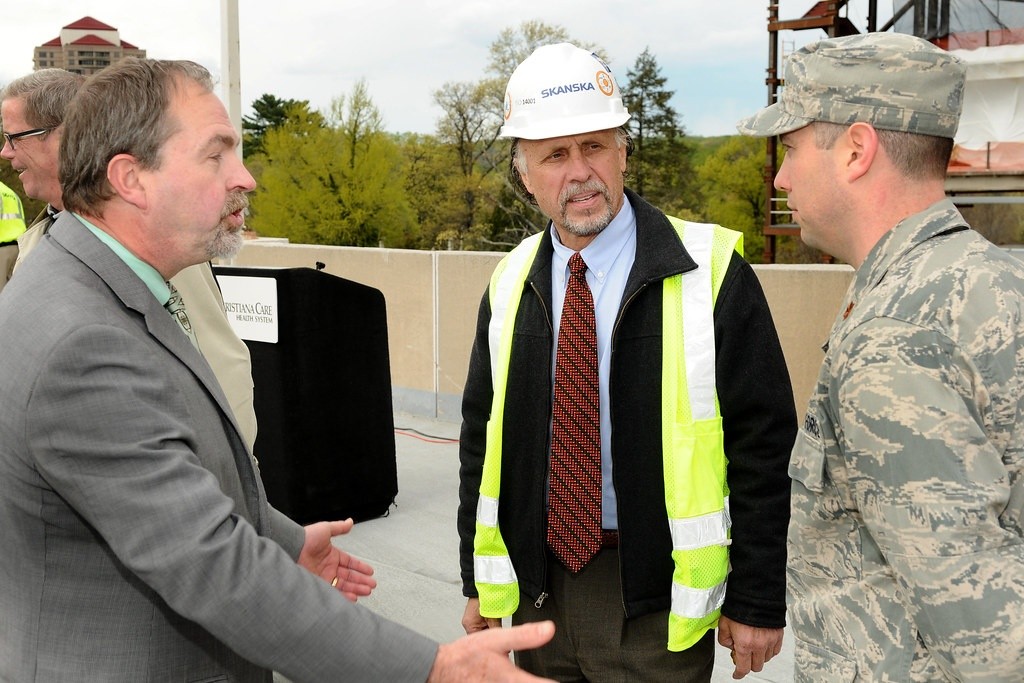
[501,41,632,140]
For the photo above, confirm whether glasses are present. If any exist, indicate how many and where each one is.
[3,126,58,150]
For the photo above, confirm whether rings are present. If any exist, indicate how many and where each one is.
[331,576,339,586]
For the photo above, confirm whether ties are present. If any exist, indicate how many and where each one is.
[165,281,201,354]
[547,252,603,572]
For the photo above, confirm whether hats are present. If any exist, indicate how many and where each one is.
[737,31,967,137]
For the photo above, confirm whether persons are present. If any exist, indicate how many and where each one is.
[457,42,799,683]
[737,32,1024,683]
[0,57,555,683]
[0,68,258,460]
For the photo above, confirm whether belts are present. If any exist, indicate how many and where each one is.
[544,526,619,545]
